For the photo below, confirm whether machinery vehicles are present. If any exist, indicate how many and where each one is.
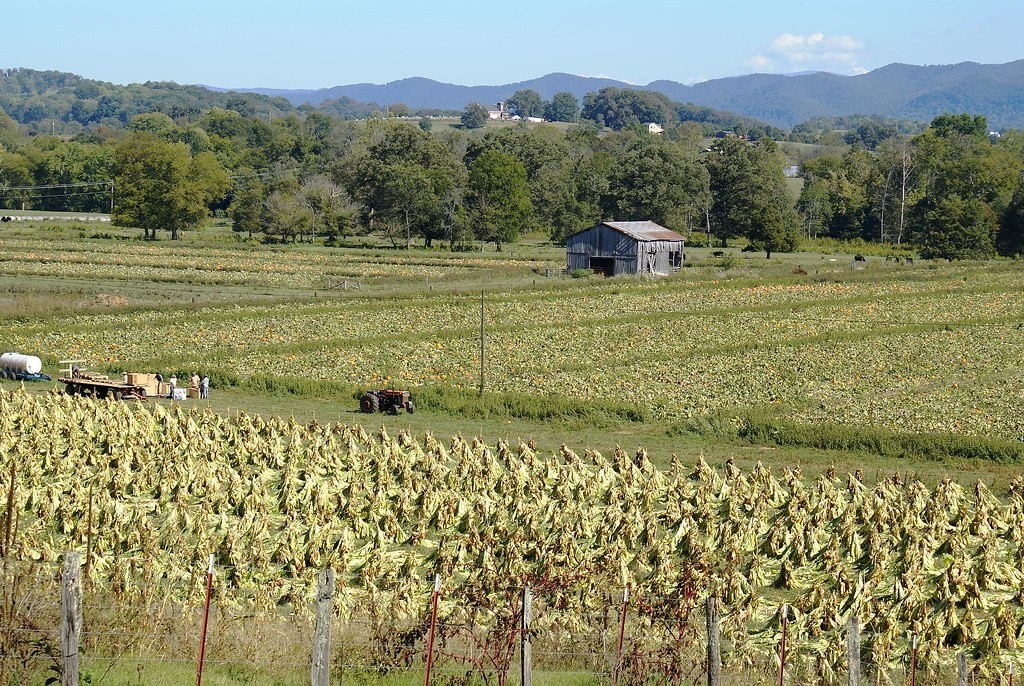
[359,379,416,416]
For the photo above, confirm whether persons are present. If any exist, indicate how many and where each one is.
[189,372,200,388]
[127,369,132,374]
[199,374,209,399]
[153,372,162,383]
[169,374,177,399]
